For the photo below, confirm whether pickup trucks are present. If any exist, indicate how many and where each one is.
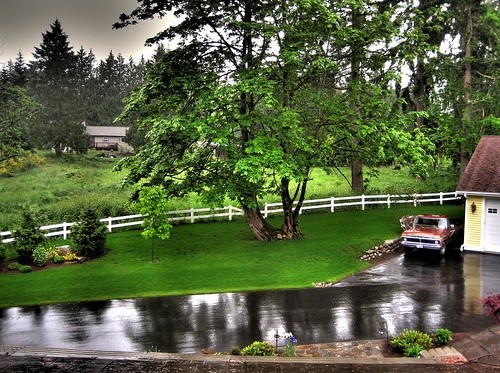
[402,213,464,255]
